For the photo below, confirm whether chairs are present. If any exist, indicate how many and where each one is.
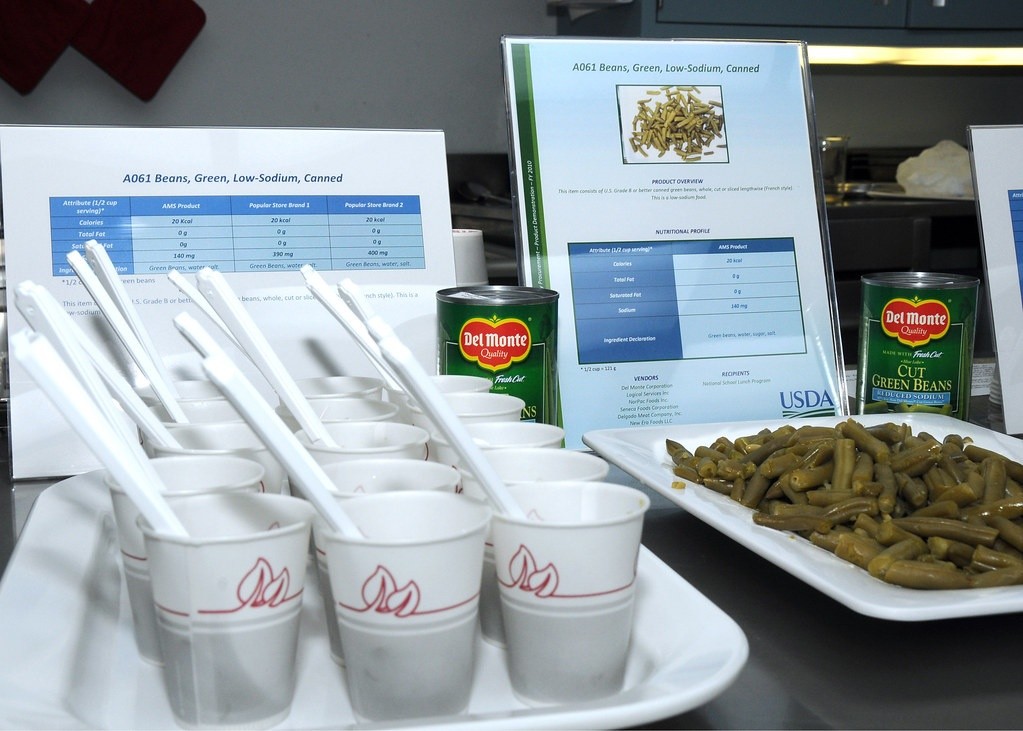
[824,215,932,332]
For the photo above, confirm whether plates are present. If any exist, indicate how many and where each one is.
[580,412,1023,622]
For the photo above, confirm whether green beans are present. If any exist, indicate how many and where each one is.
[664,418,1023,590]
[862,401,953,416]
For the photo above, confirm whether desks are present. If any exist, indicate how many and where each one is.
[827,200,980,273]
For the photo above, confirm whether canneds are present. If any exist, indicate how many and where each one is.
[857,271,980,423]
[437,284,560,426]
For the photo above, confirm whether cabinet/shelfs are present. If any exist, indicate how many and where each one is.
[557,0,1023,47]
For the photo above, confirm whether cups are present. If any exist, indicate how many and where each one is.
[98,372,653,730]
